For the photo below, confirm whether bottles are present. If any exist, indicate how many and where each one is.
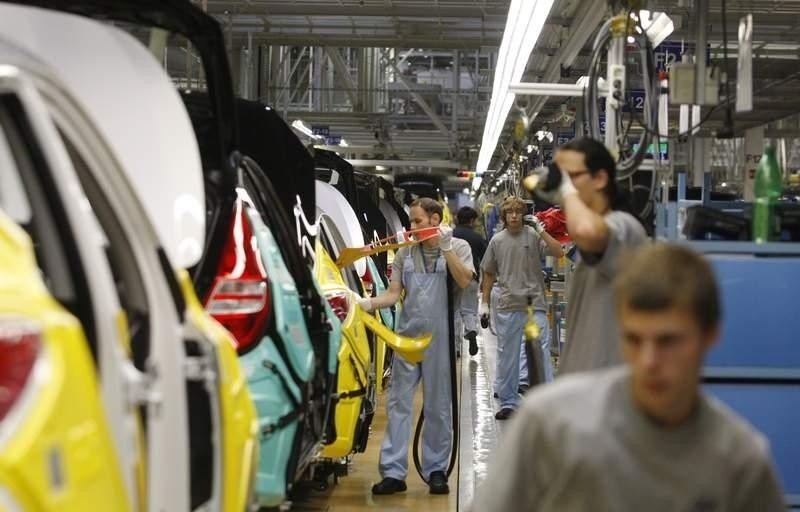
[750,143,784,245]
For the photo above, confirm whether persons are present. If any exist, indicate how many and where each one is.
[480,196,566,421]
[339,195,478,496]
[492,213,557,400]
[446,205,493,359]
[457,233,795,510]
[523,136,650,380]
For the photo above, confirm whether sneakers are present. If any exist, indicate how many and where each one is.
[371,478,407,495]
[429,471,450,495]
[469,332,478,356]
[494,407,515,421]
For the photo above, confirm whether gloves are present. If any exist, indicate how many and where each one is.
[437,223,453,252]
[530,168,576,204]
[479,303,489,329]
[522,214,544,235]
